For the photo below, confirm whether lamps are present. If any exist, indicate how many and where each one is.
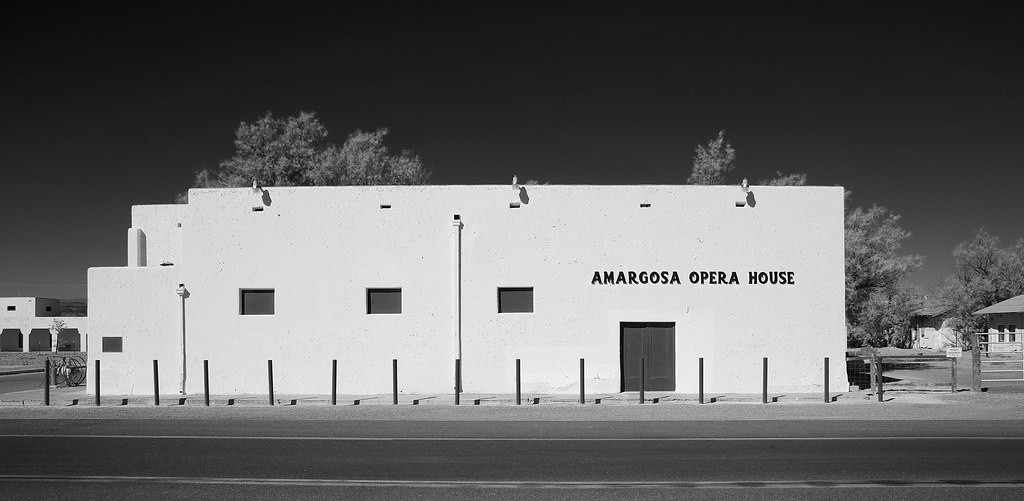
[512,174,519,185]
[742,179,749,188]
[252,180,258,190]
